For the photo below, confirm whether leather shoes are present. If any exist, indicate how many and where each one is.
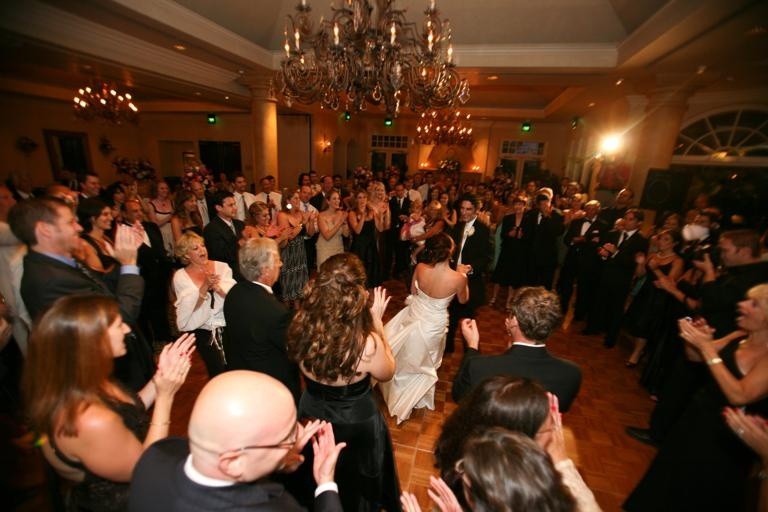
[579,324,617,348]
[624,426,661,448]
[489,298,497,306]
[627,356,641,368]
[507,305,511,312]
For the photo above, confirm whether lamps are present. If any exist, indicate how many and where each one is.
[72,70,138,124]
[267,0,471,117]
[414,117,475,147]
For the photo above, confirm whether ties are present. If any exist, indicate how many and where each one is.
[241,196,250,218]
[618,232,628,247]
[267,195,272,221]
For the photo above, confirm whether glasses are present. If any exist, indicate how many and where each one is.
[221,424,298,455]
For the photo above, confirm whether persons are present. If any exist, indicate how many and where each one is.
[1,153,768,447]
[397,424,579,511]
[431,374,604,512]
[618,284,768,511]
[721,404,768,512]
[128,369,351,512]
[18,292,198,511]
[283,250,406,512]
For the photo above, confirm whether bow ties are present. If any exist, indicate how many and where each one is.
[582,218,592,225]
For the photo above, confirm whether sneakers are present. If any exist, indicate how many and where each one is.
[410,254,417,266]
